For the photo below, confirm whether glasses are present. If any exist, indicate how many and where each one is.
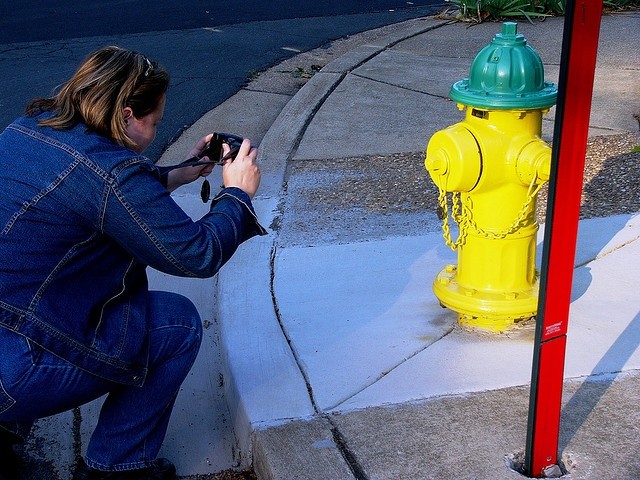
[129,57,154,102]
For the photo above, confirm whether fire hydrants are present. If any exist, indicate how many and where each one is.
[423,21,557,336]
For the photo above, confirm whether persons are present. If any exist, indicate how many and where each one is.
[0,45,269,480]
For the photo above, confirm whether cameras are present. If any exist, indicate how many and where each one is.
[207,132,245,165]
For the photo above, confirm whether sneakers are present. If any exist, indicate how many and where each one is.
[1,452,27,476]
[72,458,177,478]
